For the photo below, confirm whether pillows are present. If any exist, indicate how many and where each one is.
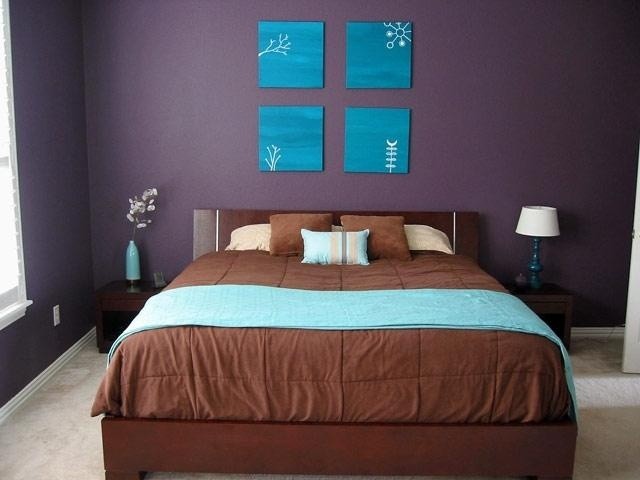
[301,228,371,266]
[340,214,412,260]
[269,213,333,257]
[228,222,452,255]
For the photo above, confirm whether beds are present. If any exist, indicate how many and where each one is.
[91,209,580,480]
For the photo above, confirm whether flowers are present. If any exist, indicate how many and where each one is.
[126,188,158,240]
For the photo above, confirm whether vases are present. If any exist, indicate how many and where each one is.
[125,240,140,280]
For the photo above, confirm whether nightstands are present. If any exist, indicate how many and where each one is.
[511,284,573,351]
[95,280,170,353]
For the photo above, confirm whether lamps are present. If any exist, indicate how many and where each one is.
[515,205,560,284]
